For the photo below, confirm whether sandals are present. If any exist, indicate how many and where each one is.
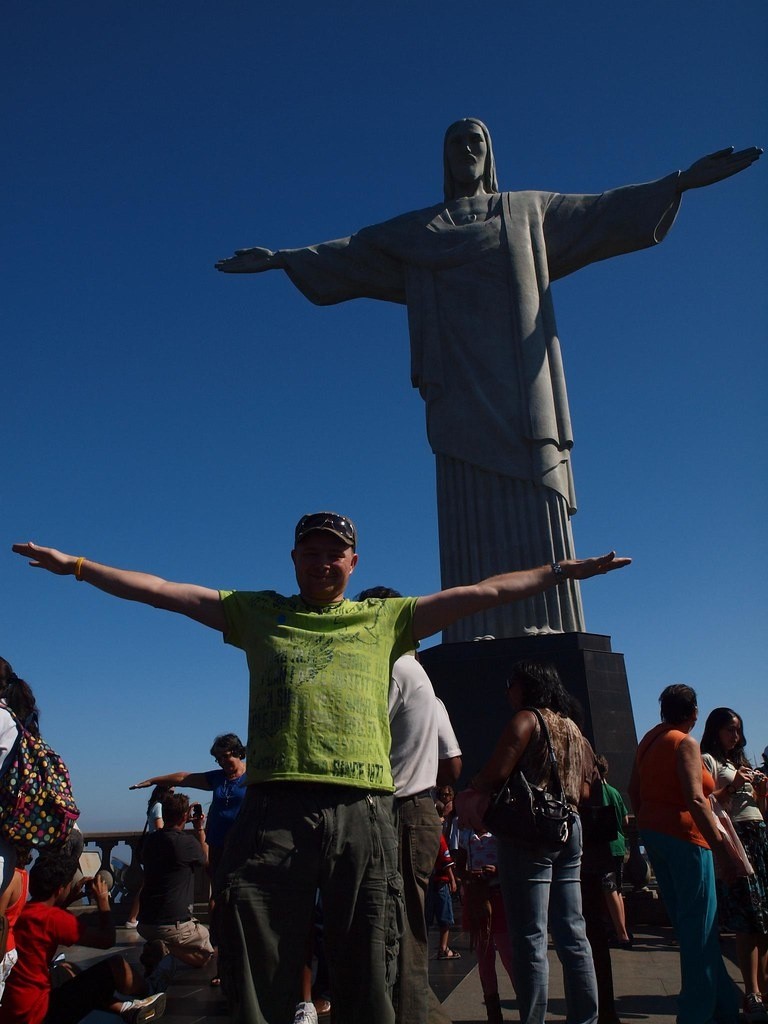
[438,947,462,959]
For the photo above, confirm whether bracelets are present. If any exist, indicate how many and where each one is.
[73,557,85,581]
[551,563,564,585]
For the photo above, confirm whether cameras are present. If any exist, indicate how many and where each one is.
[194,805,202,819]
[748,772,764,784]
[85,878,101,893]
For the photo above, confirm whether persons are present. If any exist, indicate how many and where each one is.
[14,512,632,1024]
[351,584,466,1024]
[214,119,765,641]
[431,658,768,1024]
[0,660,332,1024]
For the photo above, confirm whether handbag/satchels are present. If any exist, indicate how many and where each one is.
[482,709,577,852]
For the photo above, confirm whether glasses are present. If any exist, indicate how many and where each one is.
[215,751,234,763]
[297,513,354,541]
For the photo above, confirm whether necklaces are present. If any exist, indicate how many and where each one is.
[222,765,245,804]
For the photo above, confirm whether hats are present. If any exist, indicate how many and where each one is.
[294,513,356,552]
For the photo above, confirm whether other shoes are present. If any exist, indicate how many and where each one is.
[743,992,768,1024]
[293,1002,318,1024]
[211,976,221,985]
[122,992,167,1024]
[607,936,632,950]
[143,941,163,984]
